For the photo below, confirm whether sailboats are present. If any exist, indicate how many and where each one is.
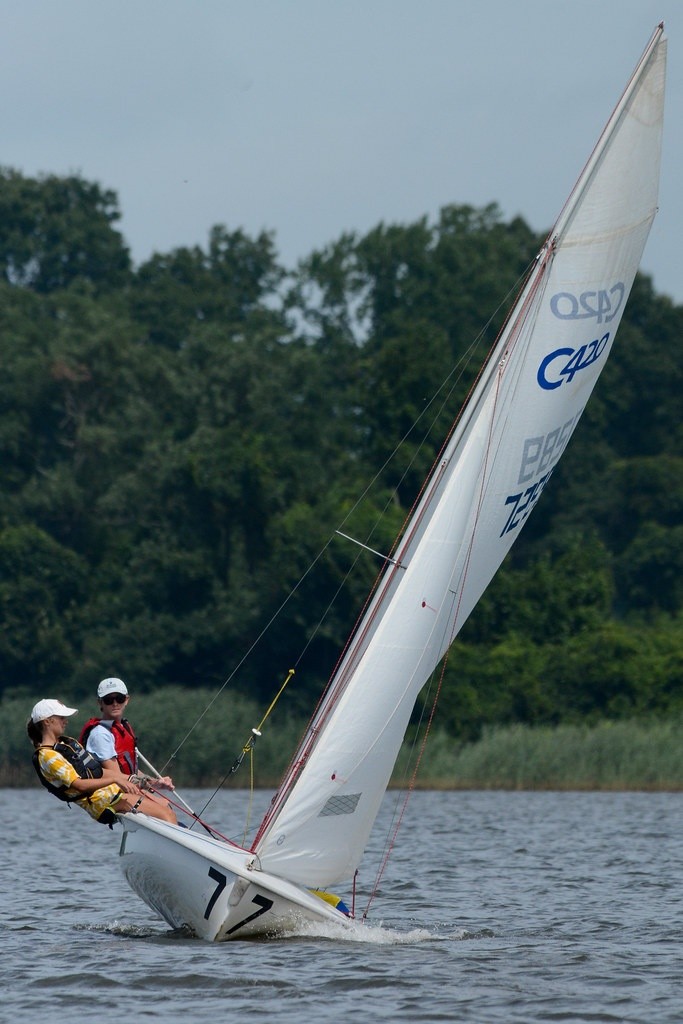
[111,21,670,941]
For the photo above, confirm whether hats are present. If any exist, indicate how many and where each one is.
[30,699,79,723]
[98,678,128,697]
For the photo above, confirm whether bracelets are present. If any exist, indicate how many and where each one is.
[127,774,133,782]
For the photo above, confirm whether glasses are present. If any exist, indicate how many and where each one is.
[101,695,126,705]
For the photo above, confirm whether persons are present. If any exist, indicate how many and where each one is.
[27,699,179,825]
[86,678,174,806]
